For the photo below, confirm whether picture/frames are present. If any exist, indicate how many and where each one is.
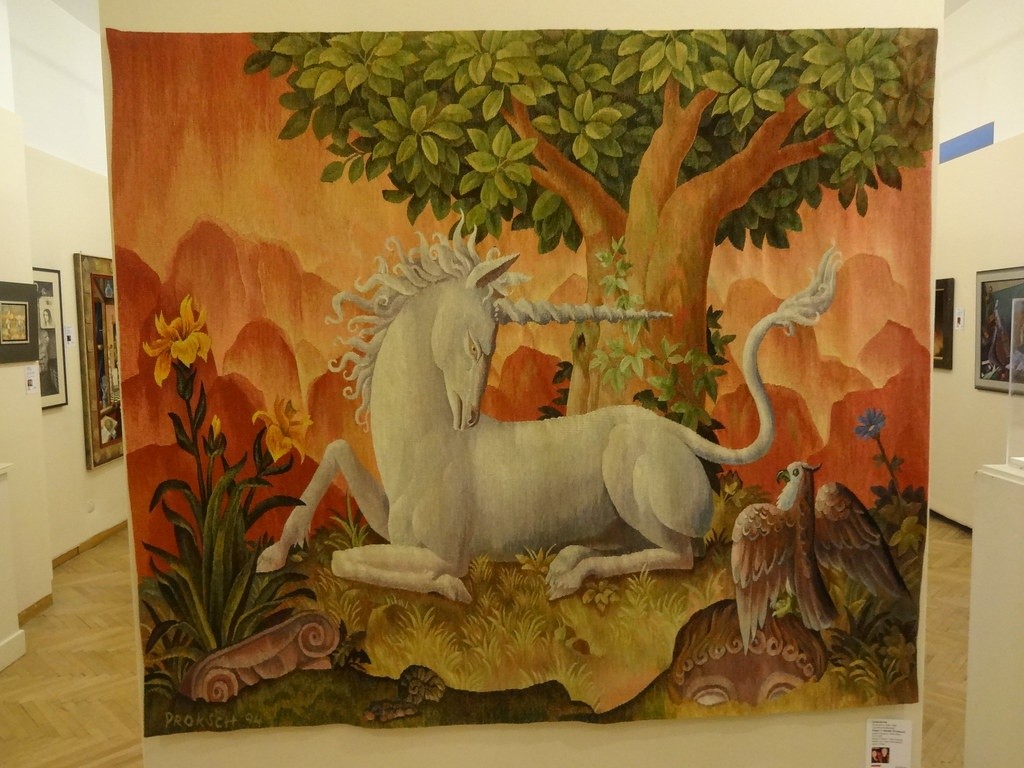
[933,277,955,370]
[32,266,68,409]
[974,265,1024,397]
[0,281,39,363]
[72,252,125,471]
